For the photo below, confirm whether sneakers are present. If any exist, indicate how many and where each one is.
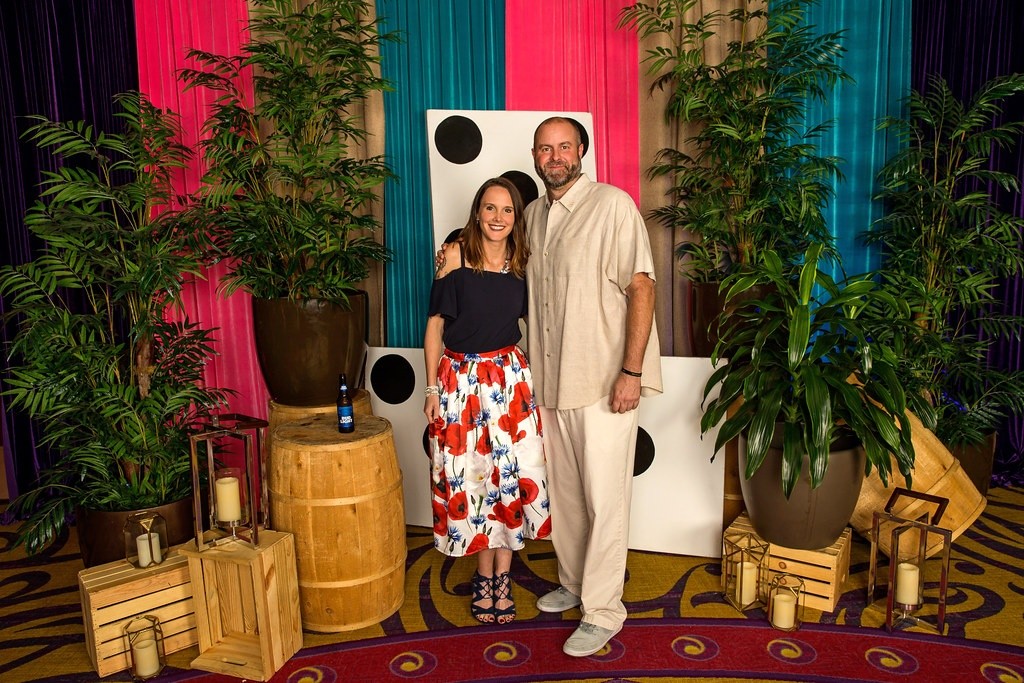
[563,621,623,657]
[536,586,581,612]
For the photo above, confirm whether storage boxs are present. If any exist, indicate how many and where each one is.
[78,543,199,677]
[721,512,852,615]
[178,525,306,682]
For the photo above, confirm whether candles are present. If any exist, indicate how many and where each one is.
[896,561,919,605]
[771,594,796,629]
[136,533,162,566]
[210,466,246,528]
[735,562,756,605]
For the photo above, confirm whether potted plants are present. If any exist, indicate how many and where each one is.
[618,1,1024,550]
[0,91,213,567]
[187,0,412,408]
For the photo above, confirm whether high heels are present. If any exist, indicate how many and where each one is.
[471,568,496,624]
[493,571,516,625]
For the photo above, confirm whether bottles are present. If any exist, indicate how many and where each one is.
[336,373,354,434]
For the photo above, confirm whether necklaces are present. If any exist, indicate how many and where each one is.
[483,244,511,275]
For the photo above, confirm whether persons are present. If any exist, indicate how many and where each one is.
[434,115,666,658]
[422,176,553,625]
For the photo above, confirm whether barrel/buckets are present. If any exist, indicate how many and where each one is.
[274,413,407,633]
[828,371,987,564]
[268,389,373,530]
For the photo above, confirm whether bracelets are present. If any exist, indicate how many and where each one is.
[621,366,642,378]
[424,385,440,398]
